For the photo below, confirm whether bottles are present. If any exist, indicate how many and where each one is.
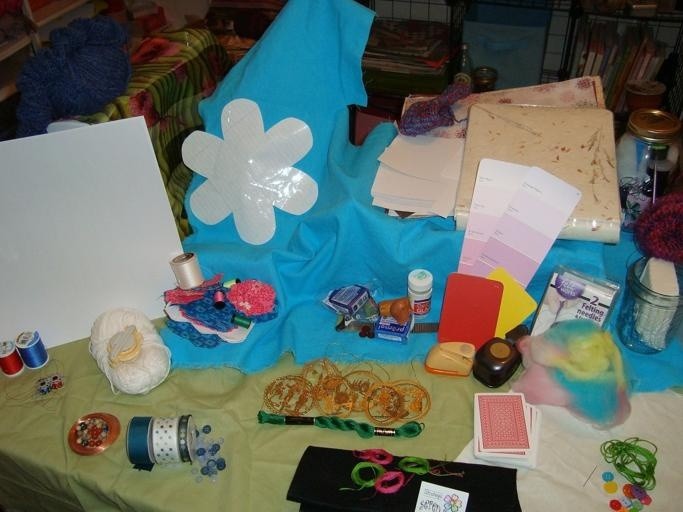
[457,43,476,94]
[615,107,683,232]
[407,269,434,315]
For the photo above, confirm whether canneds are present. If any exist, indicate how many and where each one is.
[617,107,683,212]
[471,65,498,94]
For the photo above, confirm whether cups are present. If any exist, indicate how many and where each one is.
[615,256,683,355]
[624,80,666,107]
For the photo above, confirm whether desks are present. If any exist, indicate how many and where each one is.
[203,7,459,119]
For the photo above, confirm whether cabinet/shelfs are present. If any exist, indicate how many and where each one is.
[0,0,109,104]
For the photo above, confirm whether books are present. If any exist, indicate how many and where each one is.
[362,17,457,77]
[563,15,666,115]
